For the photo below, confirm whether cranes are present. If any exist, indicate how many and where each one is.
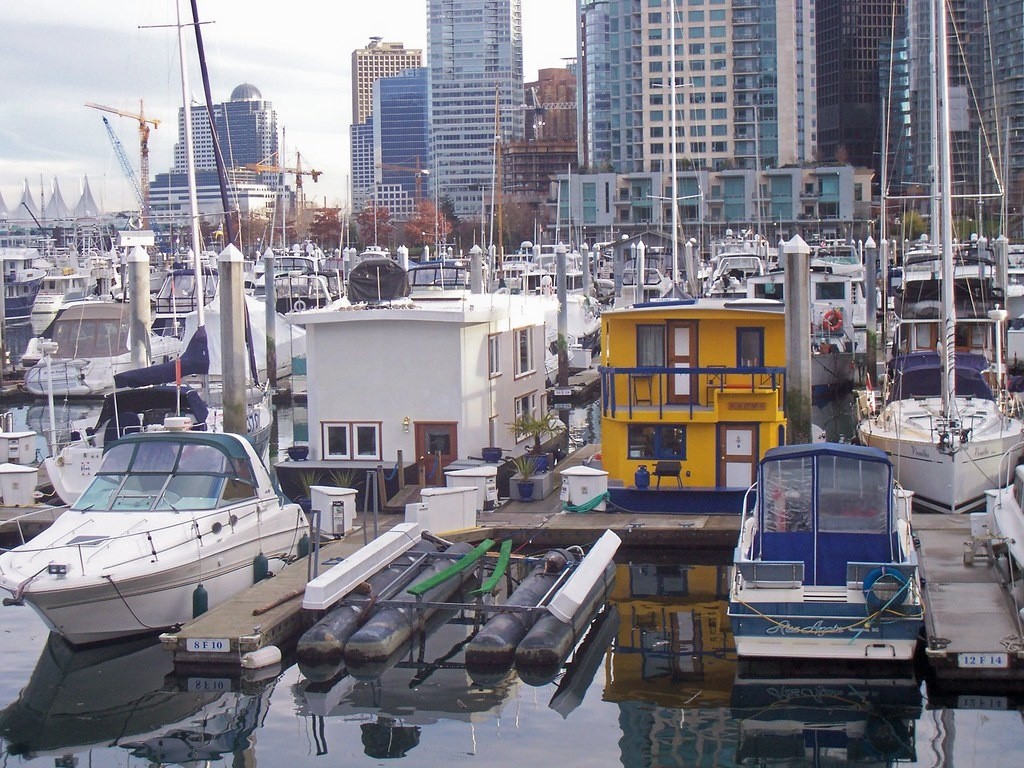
[245,124,324,238]
[102,114,171,255]
[372,154,429,218]
[82,97,162,233]
[504,83,579,142]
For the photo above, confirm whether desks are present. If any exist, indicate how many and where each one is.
[631,375,653,406]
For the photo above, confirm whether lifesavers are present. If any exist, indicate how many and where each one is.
[860,567,909,610]
[823,311,842,332]
[294,300,307,314]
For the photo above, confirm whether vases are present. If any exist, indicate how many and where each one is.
[481,447,502,464]
[287,446,309,462]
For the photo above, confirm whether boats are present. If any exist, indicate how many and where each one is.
[730,658,925,761]
[730,441,925,664]
[0,632,279,768]
[2,0,1023,648]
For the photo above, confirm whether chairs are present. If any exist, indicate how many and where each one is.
[758,366,781,408]
[652,461,683,489]
[706,365,726,408]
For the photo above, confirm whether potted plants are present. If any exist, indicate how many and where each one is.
[508,455,545,502]
[504,409,567,475]
[291,467,324,513]
